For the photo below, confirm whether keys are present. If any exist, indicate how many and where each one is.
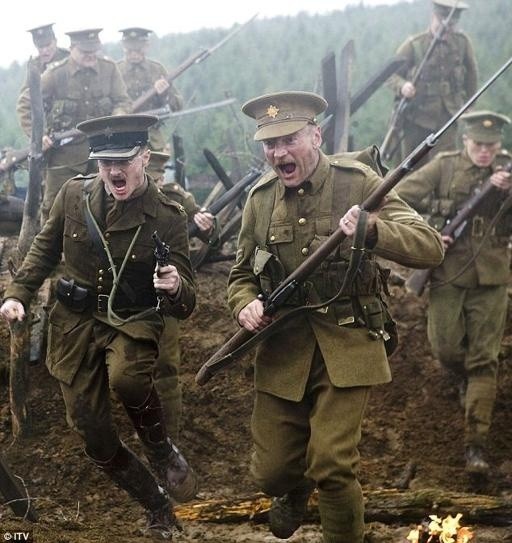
[156,295,164,312]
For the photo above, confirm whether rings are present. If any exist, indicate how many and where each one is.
[345,220,351,225]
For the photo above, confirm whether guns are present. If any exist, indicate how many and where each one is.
[196,56,511,397]
[114,11,260,118]
[406,164,510,298]
[0,98,235,172]
[380,0,461,158]
[153,231,170,297]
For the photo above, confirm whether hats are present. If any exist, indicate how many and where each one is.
[120,28,152,42]
[433,2,468,21]
[66,29,103,52]
[76,114,158,162]
[241,91,327,141]
[460,112,510,144]
[28,24,54,46]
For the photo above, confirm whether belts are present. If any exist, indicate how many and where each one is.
[87,293,131,311]
[462,215,511,238]
[267,279,333,315]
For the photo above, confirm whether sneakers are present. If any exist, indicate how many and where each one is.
[464,445,489,478]
[442,372,466,397]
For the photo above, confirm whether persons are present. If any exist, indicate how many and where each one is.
[144,154,217,443]
[16,29,134,230]
[0,116,200,541]
[21,21,71,204]
[391,110,511,474]
[115,28,184,153]
[226,92,446,542]
[385,1,479,176]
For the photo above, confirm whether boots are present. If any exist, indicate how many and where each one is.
[125,381,200,503]
[84,440,175,539]
[319,478,365,543]
[270,483,316,539]
